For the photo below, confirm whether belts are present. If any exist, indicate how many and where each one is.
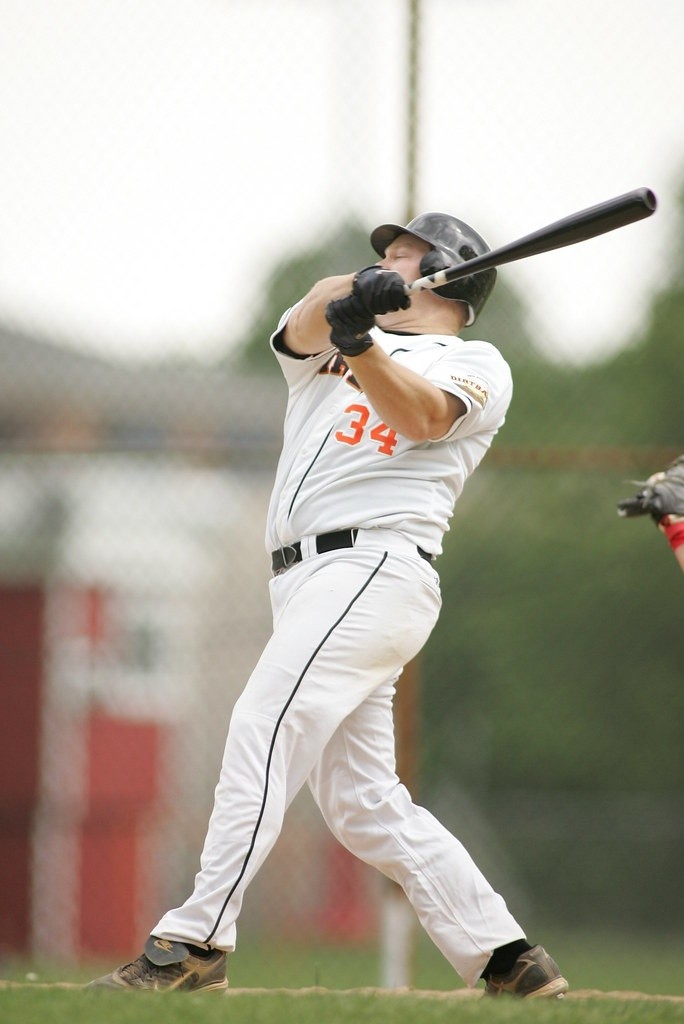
[272,529,431,571]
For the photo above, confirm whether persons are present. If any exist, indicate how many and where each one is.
[90,212,568,999]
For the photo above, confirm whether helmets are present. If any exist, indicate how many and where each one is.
[370,212,498,327]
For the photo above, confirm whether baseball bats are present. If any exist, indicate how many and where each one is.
[401,185,658,298]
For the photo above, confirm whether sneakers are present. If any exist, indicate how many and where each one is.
[476,944,569,1005]
[80,935,229,1006]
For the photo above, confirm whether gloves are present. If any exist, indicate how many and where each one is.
[351,265,411,315]
[325,295,373,357]
[643,479,684,527]
